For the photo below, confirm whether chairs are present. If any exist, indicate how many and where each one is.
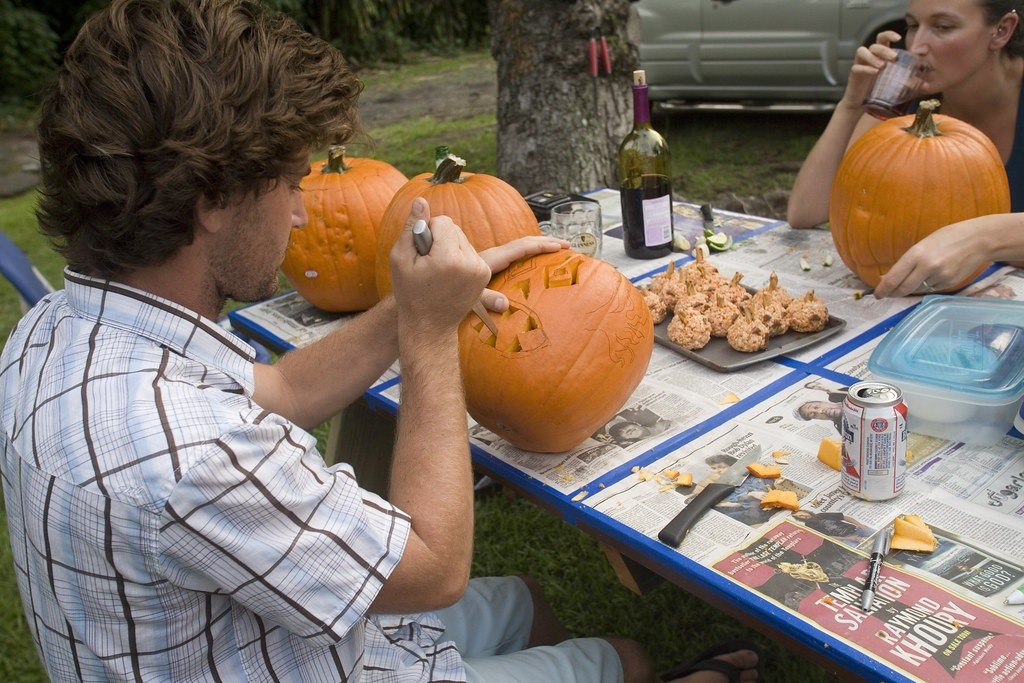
[0,231,56,308]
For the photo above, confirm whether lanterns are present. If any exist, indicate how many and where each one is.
[458,248,654,454]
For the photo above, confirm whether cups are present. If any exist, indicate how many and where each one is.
[862,45,930,120]
[552,201,602,261]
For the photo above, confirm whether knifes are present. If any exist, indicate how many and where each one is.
[700,203,714,239]
[657,445,762,547]
[413,218,499,337]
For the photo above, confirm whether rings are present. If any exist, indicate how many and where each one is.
[923,281,930,289]
[929,286,936,292]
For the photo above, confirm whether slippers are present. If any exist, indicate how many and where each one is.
[659,639,761,683]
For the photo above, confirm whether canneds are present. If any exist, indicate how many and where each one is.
[840,380,908,502]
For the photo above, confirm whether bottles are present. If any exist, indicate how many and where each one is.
[620,70,674,258]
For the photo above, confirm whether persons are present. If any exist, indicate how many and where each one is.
[791,510,877,547]
[704,454,781,525]
[604,419,671,441]
[0,0,762,683]
[792,400,842,435]
[787,0,1024,300]
[805,381,849,403]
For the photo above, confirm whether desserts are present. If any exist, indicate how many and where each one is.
[634,248,828,352]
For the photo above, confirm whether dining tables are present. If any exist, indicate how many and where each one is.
[228,186,1023,683]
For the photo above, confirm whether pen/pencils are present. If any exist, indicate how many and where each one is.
[859,527,892,613]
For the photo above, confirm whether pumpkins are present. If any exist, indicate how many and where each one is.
[827,98,1011,294]
[373,156,541,301]
[279,145,409,314]
